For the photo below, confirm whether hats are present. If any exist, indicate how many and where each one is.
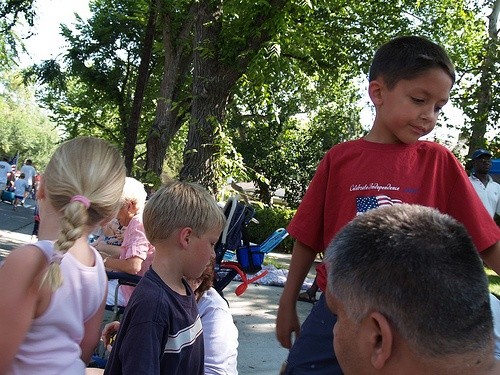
[470,149,492,159]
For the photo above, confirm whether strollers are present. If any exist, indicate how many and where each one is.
[212,195,289,295]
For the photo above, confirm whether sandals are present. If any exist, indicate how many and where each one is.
[297,290,316,303]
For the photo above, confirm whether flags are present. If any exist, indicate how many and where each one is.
[8,151,18,165]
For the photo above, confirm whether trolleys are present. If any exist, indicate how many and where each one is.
[1,187,17,205]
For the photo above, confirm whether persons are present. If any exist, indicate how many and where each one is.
[275,36,500,375]
[0,137,239,375]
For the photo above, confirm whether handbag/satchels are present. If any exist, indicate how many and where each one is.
[237,220,264,274]
